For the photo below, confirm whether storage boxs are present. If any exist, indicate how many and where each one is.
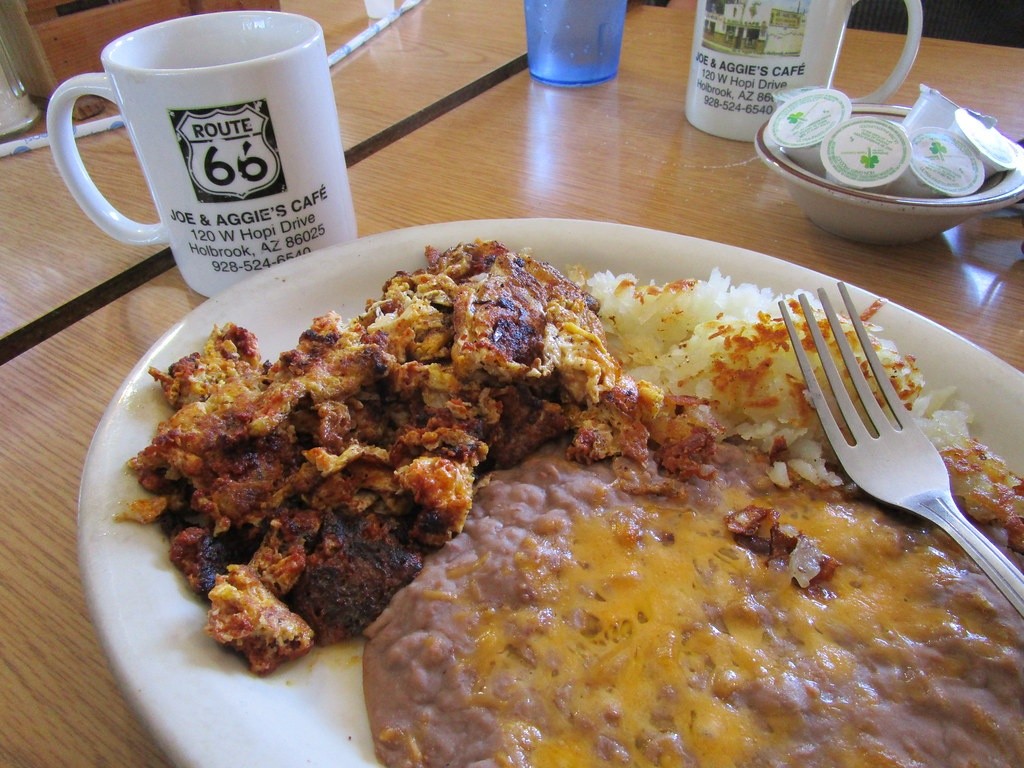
[0,0,280,121]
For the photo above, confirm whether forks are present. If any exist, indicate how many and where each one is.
[778,283,1024,620]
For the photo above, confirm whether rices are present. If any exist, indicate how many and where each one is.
[581,269,977,488]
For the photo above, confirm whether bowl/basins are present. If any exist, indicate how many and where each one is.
[754,104,1024,243]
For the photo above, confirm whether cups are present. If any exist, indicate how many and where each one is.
[0,30,42,141]
[523,0,628,88]
[46,10,358,298]
[685,0,923,142]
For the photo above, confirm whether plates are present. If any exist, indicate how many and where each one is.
[77,219,1024,768]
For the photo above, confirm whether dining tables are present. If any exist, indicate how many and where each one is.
[0,1,1024,767]
